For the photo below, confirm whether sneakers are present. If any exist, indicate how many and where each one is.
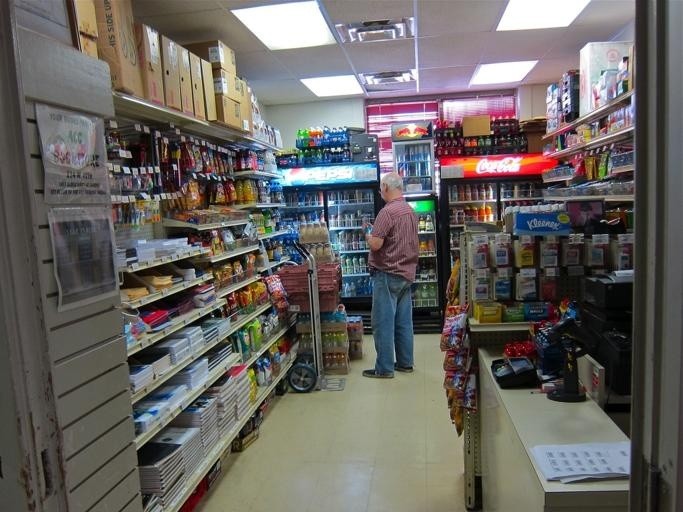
[363,370,394,378]
[394,362,413,372]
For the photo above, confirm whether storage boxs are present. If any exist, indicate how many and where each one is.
[74,1,252,137]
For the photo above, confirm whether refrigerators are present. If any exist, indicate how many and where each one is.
[440,153,536,332]
[278,163,379,332]
[394,191,446,334]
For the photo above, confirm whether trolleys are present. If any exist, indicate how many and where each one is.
[287,241,345,393]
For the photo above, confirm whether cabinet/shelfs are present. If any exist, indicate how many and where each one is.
[104,90,300,512]
[477,346,631,512]
[539,88,634,201]
[460,231,633,332]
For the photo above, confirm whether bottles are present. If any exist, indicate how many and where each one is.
[283,186,324,207]
[412,283,437,305]
[276,143,352,164]
[505,201,542,207]
[435,136,528,154]
[449,181,494,202]
[343,277,373,296]
[415,257,437,281]
[450,230,460,248]
[491,118,520,137]
[417,213,436,232]
[338,255,368,275]
[331,230,372,251]
[434,119,463,136]
[305,242,336,263]
[327,186,373,207]
[417,238,435,256]
[233,120,286,266]
[292,126,350,148]
[450,204,495,223]
[502,182,540,196]
[327,209,375,227]
[300,221,330,244]
[396,145,430,161]
[399,162,432,176]
[285,210,326,225]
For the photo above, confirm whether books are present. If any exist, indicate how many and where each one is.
[130,320,254,496]
[117,236,196,269]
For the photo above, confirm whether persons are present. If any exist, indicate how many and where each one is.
[363,172,419,379]
